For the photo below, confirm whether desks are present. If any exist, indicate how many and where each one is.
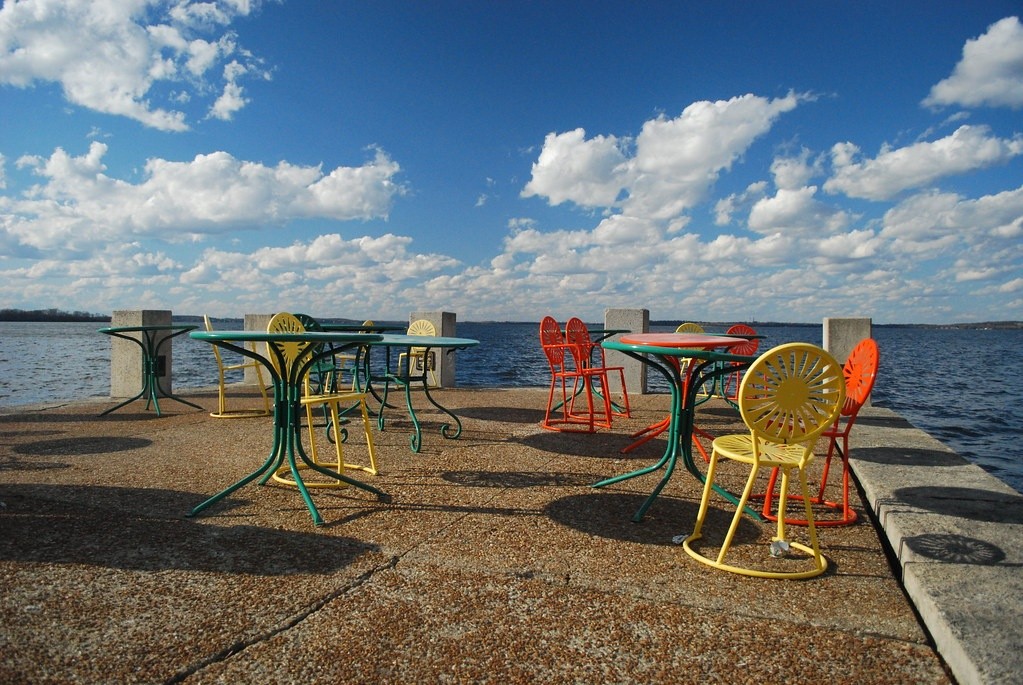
[184,324,481,526]
[591,329,767,524]
[543,328,630,414]
[95,323,206,418]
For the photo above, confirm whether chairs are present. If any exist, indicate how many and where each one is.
[285,314,335,424]
[204,313,272,419]
[683,341,846,579]
[324,319,375,393]
[540,315,612,435]
[675,322,720,399]
[567,317,632,422]
[262,312,379,488]
[723,324,770,400]
[745,336,879,527]
[395,319,437,389]
